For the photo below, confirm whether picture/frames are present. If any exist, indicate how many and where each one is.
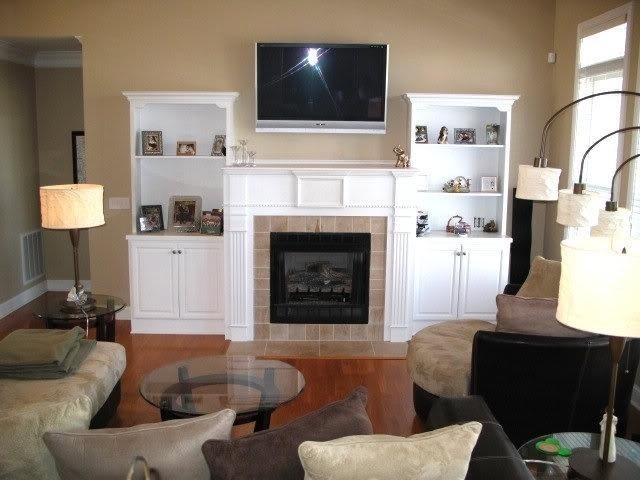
[454,128,476,145]
[177,141,195,156]
[211,135,226,156]
[201,211,223,236]
[141,131,163,156]
[480,176,499,192]
[167,195,202,233]
[137,204,164,233]
[71,131,86,183]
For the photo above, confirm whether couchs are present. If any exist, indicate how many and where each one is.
[419,394,536,480]
[406,255,640,445]
[0,328,128,432]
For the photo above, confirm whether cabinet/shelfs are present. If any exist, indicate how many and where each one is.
[122,91,241,333]
[231,164,398,214]
[409,94,512,323]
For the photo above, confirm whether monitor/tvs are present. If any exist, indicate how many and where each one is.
[253,43,390,136]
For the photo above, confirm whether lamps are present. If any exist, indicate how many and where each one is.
[515,88,639,202]
[556,126,640,228]
[38,183,105,312]
[590,153,640,237]
[555,237,640,480]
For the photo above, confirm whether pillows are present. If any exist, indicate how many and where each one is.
[515,255,562,298]
[0,393,93,479]
[200,384,374,480]
[42,409,237,480]
[296,420,482,480]
[494,294,596,337]
